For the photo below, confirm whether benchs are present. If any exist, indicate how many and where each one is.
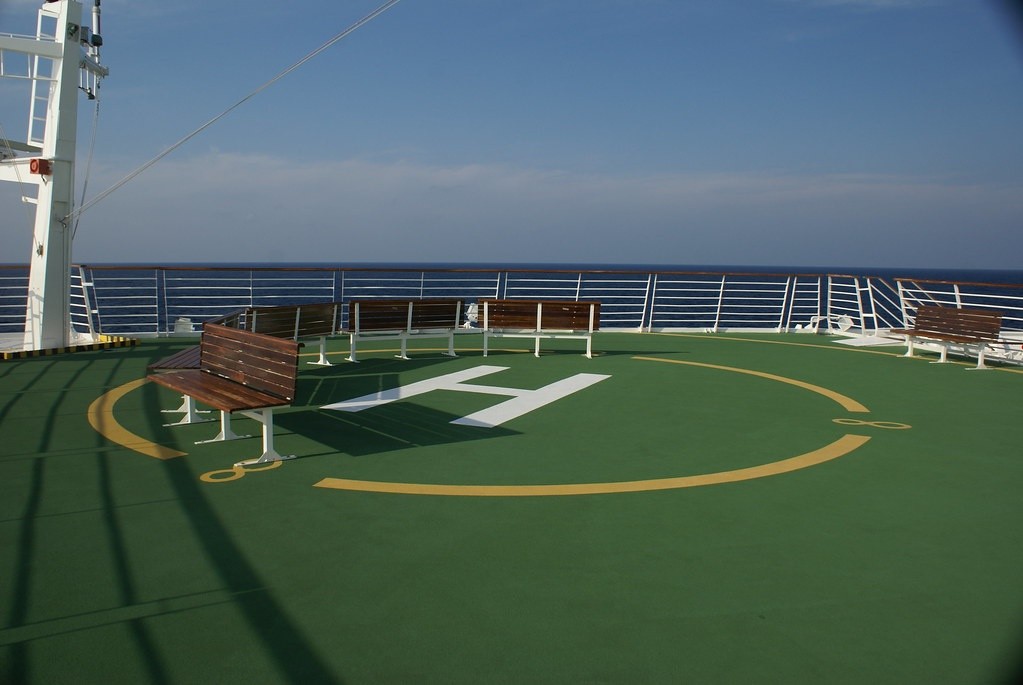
[890,307,1003,370]
[342,296,467,363]
[476,300,601,358]
[145,324,306,467]
[147,307,248,414]
[247,300,343,366]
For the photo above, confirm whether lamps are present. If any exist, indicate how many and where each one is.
[838,316,855,331]
